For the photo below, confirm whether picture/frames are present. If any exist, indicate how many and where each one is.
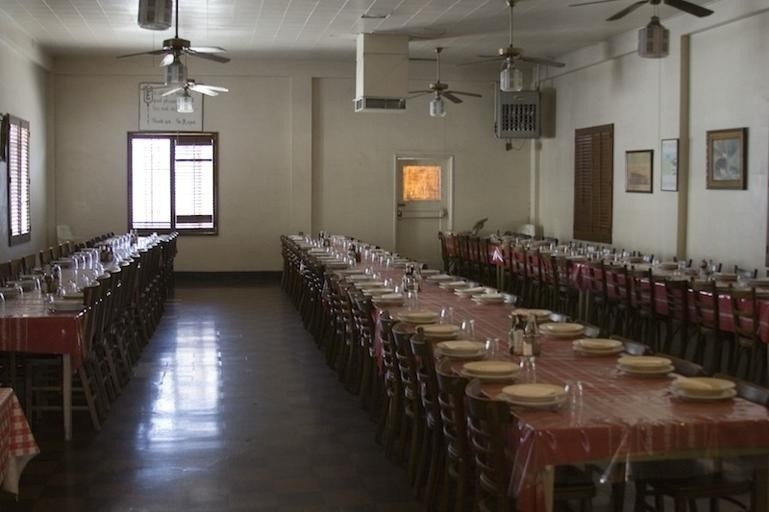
[659,138,678,192]
[705,127,747,189]
[625,150,653,192]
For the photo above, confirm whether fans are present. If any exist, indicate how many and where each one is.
[407,46,484,105]
[456,0,567,69]
[114,0,230,68]
[152,73,227,99]
[569,0,715,20]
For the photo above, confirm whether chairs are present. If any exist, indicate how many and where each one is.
[2,233,177,431]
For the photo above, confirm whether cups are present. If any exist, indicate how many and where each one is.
[439,305,499,354]
[539,243,754,288]
[519,356,583,399]
[65,231,159,298]
[40,282,75,311]
[305,227,427,308]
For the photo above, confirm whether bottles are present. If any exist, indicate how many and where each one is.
[126,228,140,243]
[95,243,114,261]
[507,312,543,356]
[400,261,425,293]
[31,263,63,294]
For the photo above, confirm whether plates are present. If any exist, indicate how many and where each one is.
[393,256,508,307]
[571,338,626,354]
[308,248,404,307]
[497,382,569,408]
[413,322,461,339]
[507,308,553,319]
[433,341,487,356]
[665,377,739,400]
[535,323,584,336]
[457,359,521,379]
[389,309,441,324]
[613,355,675,373]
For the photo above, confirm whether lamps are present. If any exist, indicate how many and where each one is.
[500,59,523,91]
[635,4,670,58]
[136,0,172,30]
[429,97,446,117]
[163,61,188,84]
[176,90,193,113]
